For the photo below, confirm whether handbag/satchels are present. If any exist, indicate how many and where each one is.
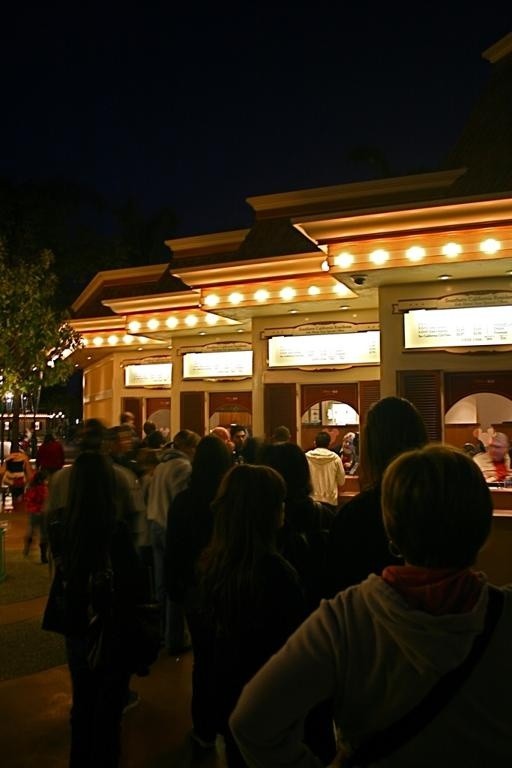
[81,570,162,677]
[13,477,25,488]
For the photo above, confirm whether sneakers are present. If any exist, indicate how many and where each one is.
[124,691,140,711]
[170,637,191,658]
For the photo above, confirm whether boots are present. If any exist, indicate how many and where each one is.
[23,538,48,565]
